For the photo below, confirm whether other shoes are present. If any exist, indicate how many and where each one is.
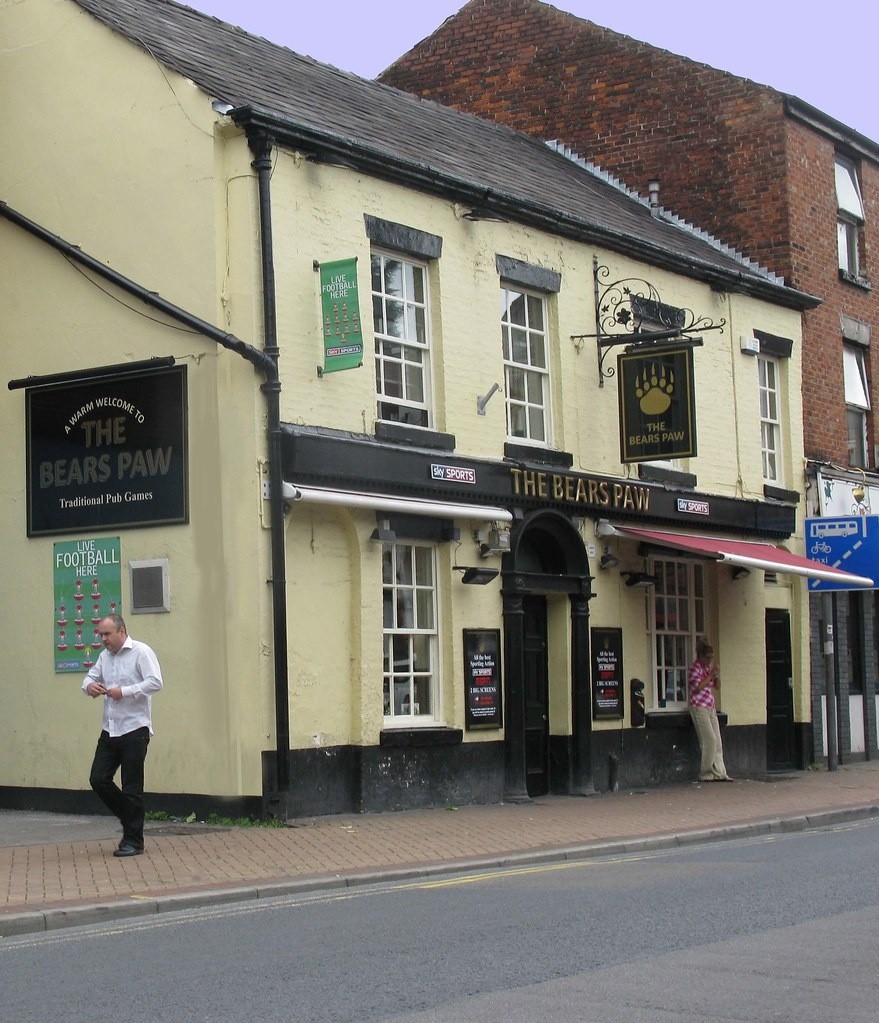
[700,775,733,782]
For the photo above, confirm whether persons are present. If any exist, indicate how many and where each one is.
[687,642,735,783]
[81,614,164,854]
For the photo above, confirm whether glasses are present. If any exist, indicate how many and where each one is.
[700,653,714,659]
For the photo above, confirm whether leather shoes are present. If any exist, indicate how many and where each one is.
[113,843,142,856]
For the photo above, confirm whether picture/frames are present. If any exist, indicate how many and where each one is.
[591,626,624,720]
[462,627,503,731]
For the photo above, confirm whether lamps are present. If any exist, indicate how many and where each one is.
[461,206,510,225]
[849,466,866,503]
[473,527,504,558]
[621,570,659,588]
[732,566,751,581]
[600,554,618,570]
[711,283,751,297]
